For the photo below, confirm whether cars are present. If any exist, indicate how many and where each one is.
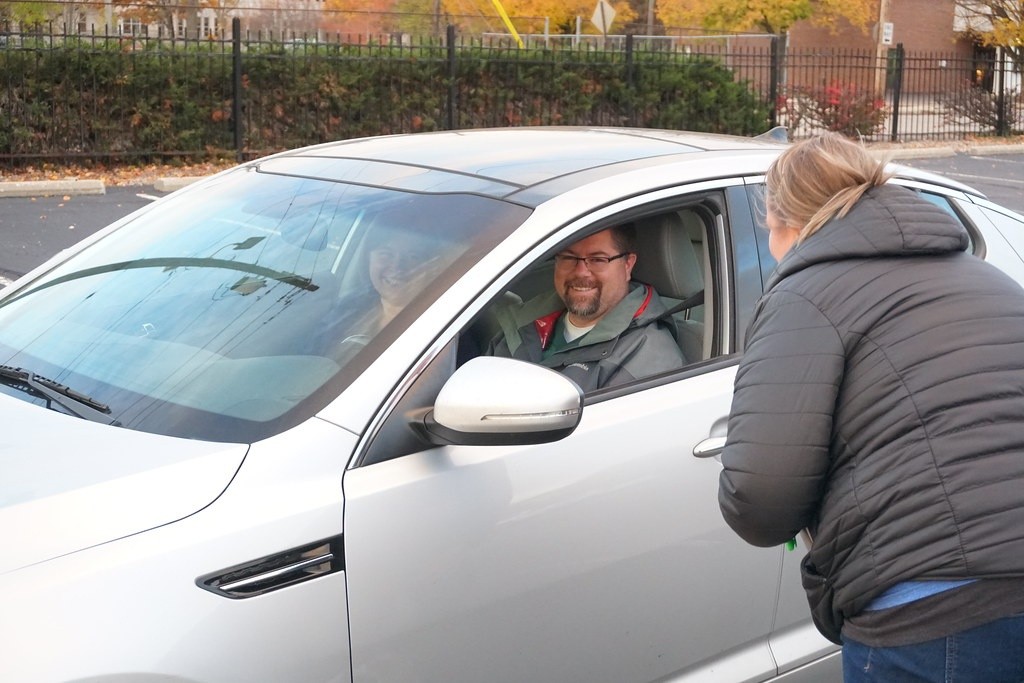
[0,130,1024,683]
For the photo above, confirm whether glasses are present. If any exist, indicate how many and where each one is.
[554,252,630,272]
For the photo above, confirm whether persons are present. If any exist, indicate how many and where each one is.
[719,133,1021,683]
[484,221,691,392]
[314,223,479,368]
